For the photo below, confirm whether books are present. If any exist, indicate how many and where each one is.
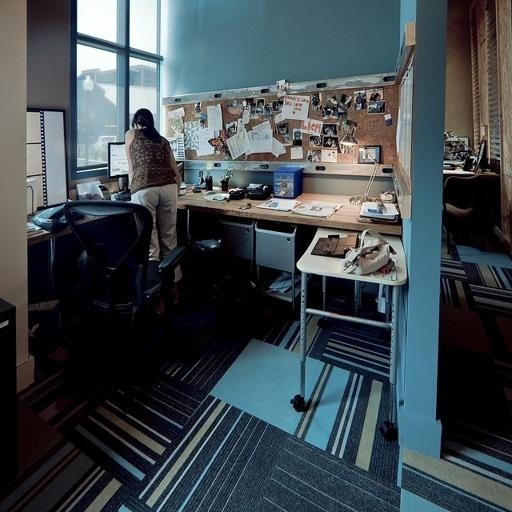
[359,201,398,223]
[293,200,343,217]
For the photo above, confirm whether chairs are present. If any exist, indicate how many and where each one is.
[443,174,501,255]
[63,201,187,316]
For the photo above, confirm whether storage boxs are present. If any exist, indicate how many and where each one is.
[272,167,305,199]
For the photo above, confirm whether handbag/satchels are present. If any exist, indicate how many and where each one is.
[343,229,396,276]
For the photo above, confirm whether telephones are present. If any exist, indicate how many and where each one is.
[97,184,111,200]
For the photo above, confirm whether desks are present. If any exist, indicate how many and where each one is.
[27,181,402,247]
[291,227,409,441]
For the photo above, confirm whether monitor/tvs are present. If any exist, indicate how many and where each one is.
[107,142,131,191]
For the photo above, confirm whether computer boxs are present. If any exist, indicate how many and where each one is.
[0,298,17,430]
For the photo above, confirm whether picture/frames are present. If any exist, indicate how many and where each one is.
[357,145,382,164]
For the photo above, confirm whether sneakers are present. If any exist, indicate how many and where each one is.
[157,297,166,315]
[171,285,180,306]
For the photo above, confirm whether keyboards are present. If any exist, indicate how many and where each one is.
[114,192,131,202]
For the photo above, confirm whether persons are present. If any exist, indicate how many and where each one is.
[293,132,302,145]
[312,95,321,110]
[265,104,271,118]
[443,203,474,217]
[325,138,332,147]
[356,92,363,104]
[257,101,264,111]
[195,103,201,111]
[332,140,336,147]
[124,108,183,316]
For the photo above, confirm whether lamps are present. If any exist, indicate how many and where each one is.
[339,127,381,206]
